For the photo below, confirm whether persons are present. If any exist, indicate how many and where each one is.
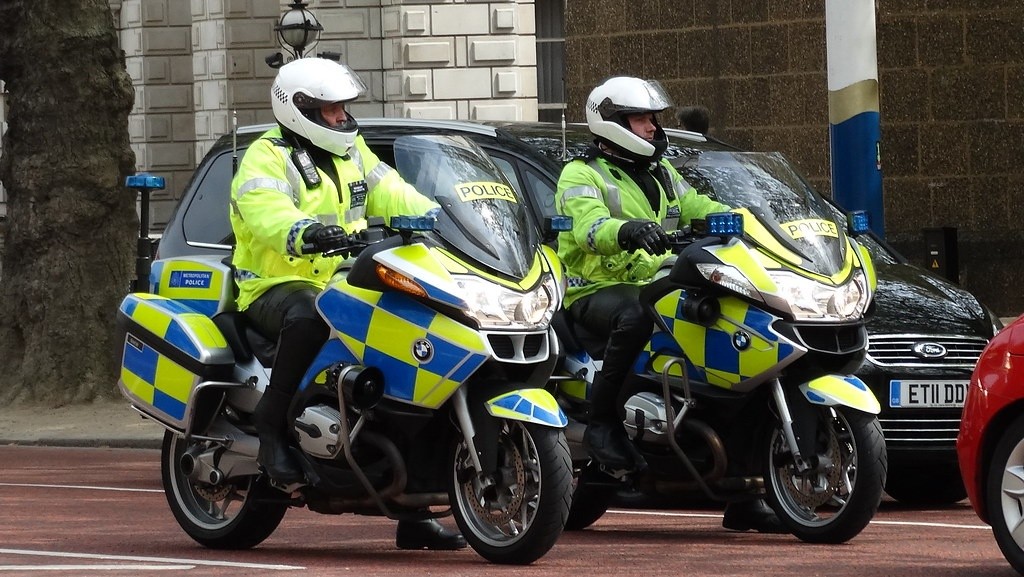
[673,103,711,134]
[230,58,467,550]
[553,76,797,532]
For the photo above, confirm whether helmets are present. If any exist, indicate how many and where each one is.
[270,58,368,160]
[587,76,671,160]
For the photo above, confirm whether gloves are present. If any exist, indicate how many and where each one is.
[618,219,672,256]
[301,222,350,258]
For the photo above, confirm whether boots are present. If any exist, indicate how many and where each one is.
[255,419,314,485]
[396,513,466,551]
[580,415,650,473]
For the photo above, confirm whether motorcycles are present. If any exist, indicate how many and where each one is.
[554,114,888,545]
[115,110,574,565]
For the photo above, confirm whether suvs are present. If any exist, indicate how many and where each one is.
[147,118,1005,512]
